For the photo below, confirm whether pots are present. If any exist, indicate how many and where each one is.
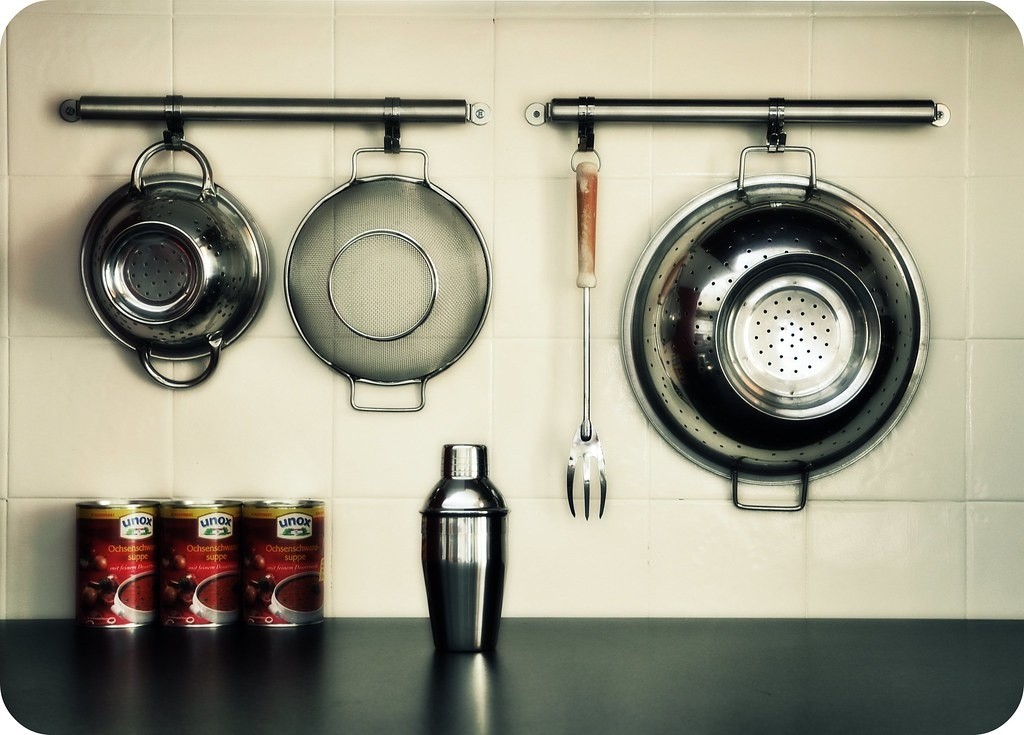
[617,144,930,513]
[282,147,491,414]
[77,139,270,390]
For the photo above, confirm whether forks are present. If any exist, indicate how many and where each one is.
[568,145,611,519]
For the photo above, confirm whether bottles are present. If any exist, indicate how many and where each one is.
[418,442,512,656]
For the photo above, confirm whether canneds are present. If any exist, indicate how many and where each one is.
[76,500,326,628]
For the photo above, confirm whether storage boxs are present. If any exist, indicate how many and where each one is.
[242,501,332,631]
[74,499,152,629]
[414,442,508,656]
[154,498,240,631]
